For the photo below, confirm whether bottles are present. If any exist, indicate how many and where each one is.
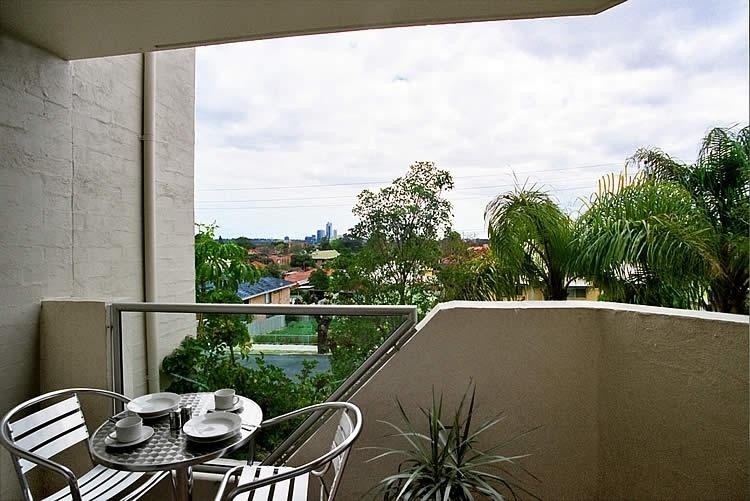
[182,406,193,425]
[168,410,181,429]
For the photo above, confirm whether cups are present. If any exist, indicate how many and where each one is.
[104,415,142,443]
[214,388,242,410]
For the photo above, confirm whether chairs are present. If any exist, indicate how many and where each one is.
[214,401,364,501]
[0,387,176,501]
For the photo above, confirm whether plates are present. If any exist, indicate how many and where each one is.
[181,412,242,444]
[105,426,155,448]
[206,403,242,413]
[124,393,182,419]
